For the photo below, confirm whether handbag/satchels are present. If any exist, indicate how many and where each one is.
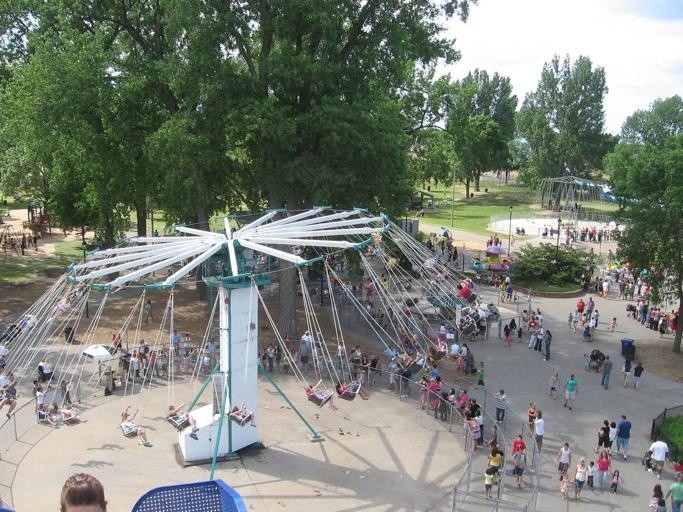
[621,367,626,373]
[597,429,604,437]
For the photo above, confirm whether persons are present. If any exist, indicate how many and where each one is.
[334,218,682,511]
[1,217,333,447]
[57,472,106,512]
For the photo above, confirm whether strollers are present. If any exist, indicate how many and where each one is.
[588,349,602,371]
[624,304,637,320]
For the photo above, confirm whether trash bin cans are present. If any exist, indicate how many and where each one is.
[65,327,75,343]
[621,337,635,360]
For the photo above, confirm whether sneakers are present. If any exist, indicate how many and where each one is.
[493,480,498,484]
[329,404,338,411]
[485,495,492,499]
[137,439,154,448]
[593,447,629,461]
[588,485,618,496]
[362,393,370,401]
[650,471,662,480]
[250,422,257,426]
[569,406,573,411]
[561,494,568,499]
[190,425,200,440]
[575,496,579,500]
[564,403,567,408]
[528,345,547,360]
